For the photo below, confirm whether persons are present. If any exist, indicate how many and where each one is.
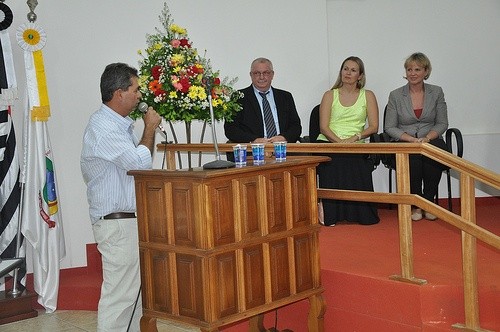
[223,58,302,156]
[383,52,449,222]
[80,62,152,331]
[315,56,381,225]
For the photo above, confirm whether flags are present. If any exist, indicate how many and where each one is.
[0,1,28,293]
[16,21,66,314]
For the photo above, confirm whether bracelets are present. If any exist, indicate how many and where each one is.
[426,134,433,142]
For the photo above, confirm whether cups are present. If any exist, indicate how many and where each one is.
[250,144,265,164]
[232,144,248,166]
[273,141,288,161]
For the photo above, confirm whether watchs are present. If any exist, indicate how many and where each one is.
[355,132,362,140]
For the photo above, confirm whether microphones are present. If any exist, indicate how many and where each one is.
[203,84,236,169]
[138,102,167,136]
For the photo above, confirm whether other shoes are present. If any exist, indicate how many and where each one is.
[412,208,422,221]
[317,203,336,226]
[424,211,436,220]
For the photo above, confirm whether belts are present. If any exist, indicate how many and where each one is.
[99,212,136,220]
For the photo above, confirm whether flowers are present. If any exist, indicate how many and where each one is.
[127,2,244,122]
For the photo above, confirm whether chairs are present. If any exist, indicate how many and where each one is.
[380,102,464,214]
[303,103,382,173]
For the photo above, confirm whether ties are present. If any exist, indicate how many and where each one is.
[259,91,277,139]
